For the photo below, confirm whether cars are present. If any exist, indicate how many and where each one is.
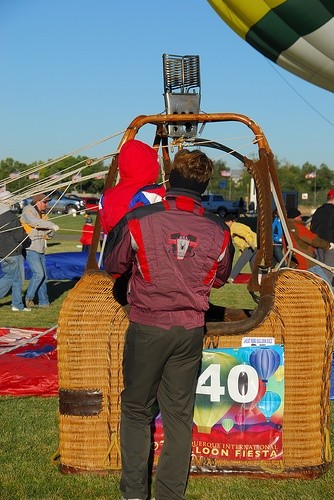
[12,189,101,216]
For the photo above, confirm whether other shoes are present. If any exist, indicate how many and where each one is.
[25,300,37,308]
[227,278,235,283]
[11,306,31,312]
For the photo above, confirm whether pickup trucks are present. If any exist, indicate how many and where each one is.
[201,194,254,219]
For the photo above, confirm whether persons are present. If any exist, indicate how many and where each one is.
[311,189,334,286]
[102,149,234,500]
[274,208,334,295]
[216,207,259,275]
[0,191,33,312]
[19,193,59,307]
[82,218,95,253]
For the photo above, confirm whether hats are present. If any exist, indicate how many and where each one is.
[287,208,301,219]
[327,189,334,198]
[0,191,15,200]
[33,194,52,203]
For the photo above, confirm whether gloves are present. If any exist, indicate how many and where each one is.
[329,242,334,249]
[54,225,60,232]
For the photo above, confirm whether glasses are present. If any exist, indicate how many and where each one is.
[43,201,47,204]
